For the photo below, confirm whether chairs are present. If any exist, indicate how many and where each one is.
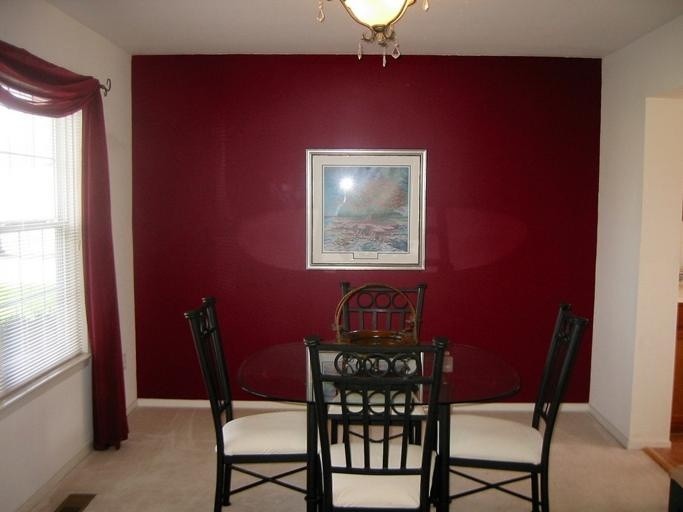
[178,293,322,512]
[336,279,429,344]
[442,298,592,512]
[303,332,450,512]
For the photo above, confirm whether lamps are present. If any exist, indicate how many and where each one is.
[312,2,433,71]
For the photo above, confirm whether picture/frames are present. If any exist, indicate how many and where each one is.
[302,144,428,272]
